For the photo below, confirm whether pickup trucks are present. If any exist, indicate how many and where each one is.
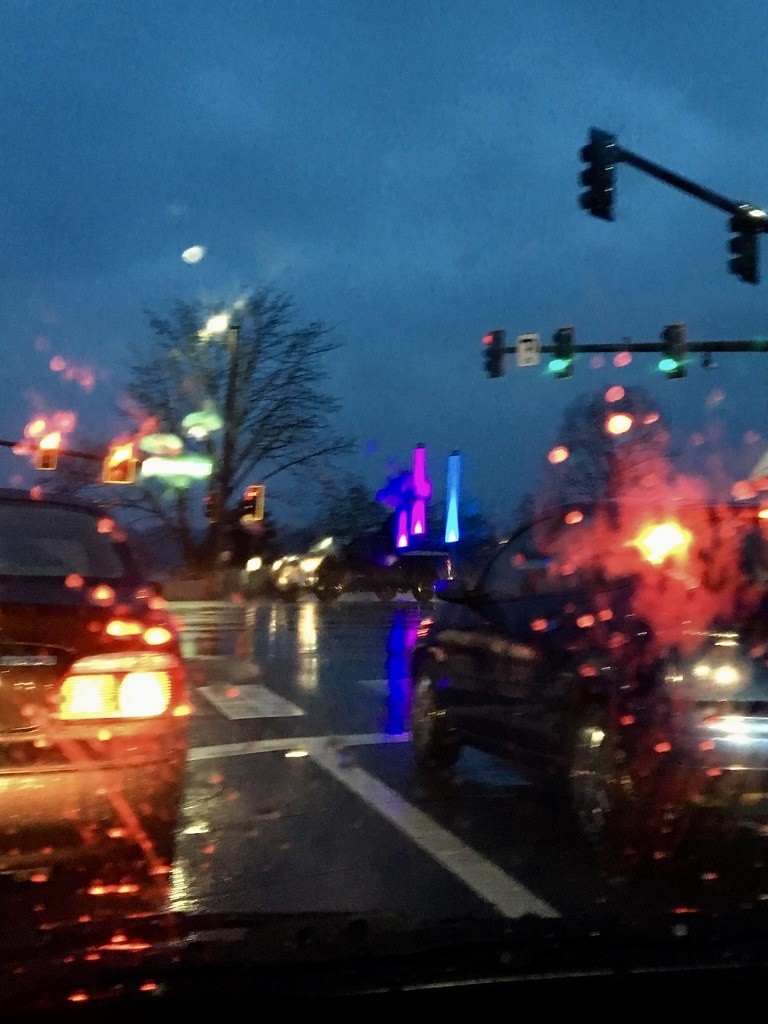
[268,533,450,605]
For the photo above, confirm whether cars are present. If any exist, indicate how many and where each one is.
[0,486,196,881]
[406,495,768,887]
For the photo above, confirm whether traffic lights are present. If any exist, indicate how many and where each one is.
[660,321,688,380]
[726,209,758,282]
[483,330,506,378]
[578,126,615,222]
[550,326,580,377]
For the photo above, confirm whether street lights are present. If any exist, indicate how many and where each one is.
[206,313,243,575]
